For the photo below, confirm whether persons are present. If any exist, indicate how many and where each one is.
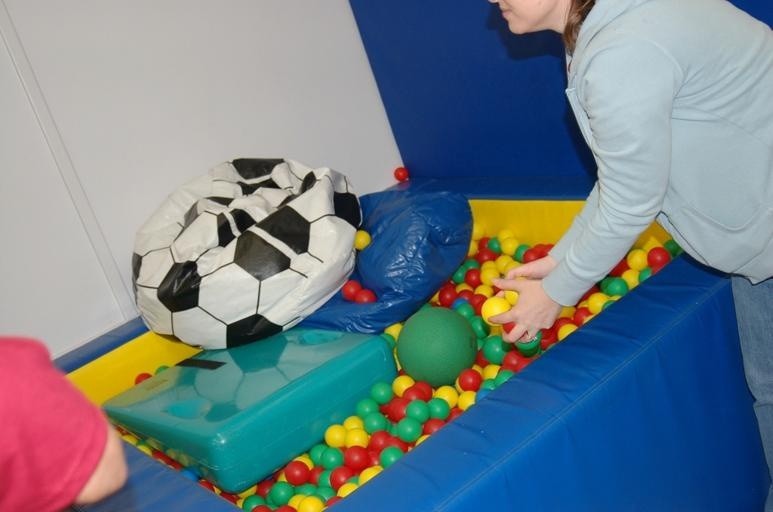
[490,1,772,512]
[0,337,129,512]
